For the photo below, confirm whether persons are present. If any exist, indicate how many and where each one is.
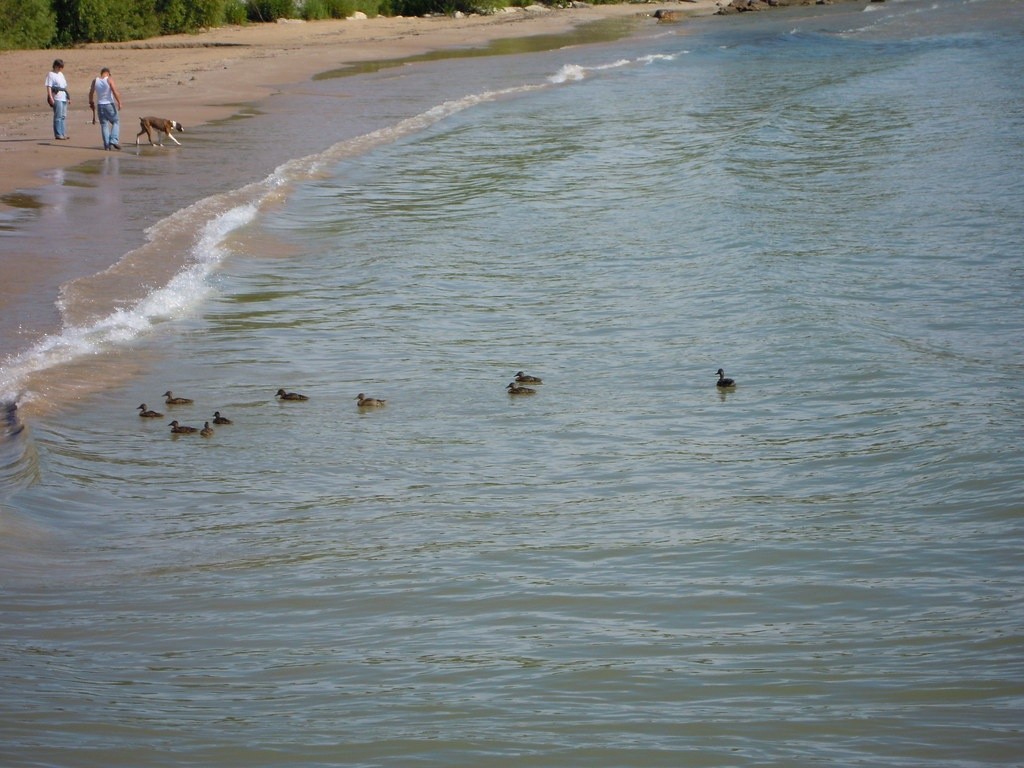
[88,68,122,150]
[44,59,72,140]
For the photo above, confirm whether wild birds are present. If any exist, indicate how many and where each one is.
[200,422,214,436]
[506,383,536,393]
[162,391,194,403]
[136,404,165,418]
[212,412,232,424]
[274,389,310,400]
[354,393,386,406]
[168,420,198,433]
[715,369,736,387]
[513,371,542,382]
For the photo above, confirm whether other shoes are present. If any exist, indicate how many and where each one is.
[54,136,70,140]
[105,143,122,151]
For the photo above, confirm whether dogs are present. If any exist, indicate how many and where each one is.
[135,117,185,147]
